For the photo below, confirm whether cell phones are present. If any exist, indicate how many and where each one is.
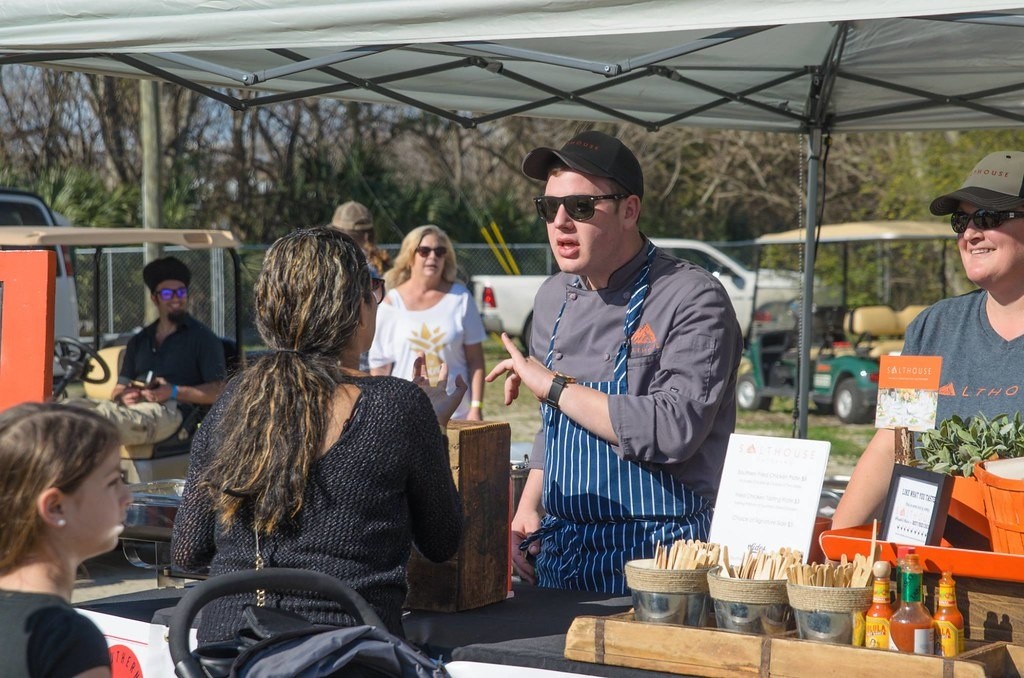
[132,370,158,390]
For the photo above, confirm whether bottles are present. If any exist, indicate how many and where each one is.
[864,560,892,652]
[932,571,965,658]
[888,554,936,656]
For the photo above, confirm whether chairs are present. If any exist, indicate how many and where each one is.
[82,346,157,458]
[810,304,932,362]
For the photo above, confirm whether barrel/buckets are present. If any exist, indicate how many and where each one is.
[973,458,1024,557]
[623,558,718,629]
[786,582,873,646]
[706,566,788,641]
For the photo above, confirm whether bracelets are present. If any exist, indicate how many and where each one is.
[170,384,177,401]
[468,400,483,409]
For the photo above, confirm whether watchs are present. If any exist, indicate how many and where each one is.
[546,371,577,410]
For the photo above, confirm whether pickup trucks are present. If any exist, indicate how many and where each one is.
[470,238,842,361]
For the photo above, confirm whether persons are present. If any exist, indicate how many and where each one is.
[0,401,131,678]
[95,257,229,446]
[166,226,464,646]
[819,147,1024,564]
[367,227,485,420]
[484,131,745,598]
[332,200,378,373]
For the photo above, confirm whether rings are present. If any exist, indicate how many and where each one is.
[155,398,159,402]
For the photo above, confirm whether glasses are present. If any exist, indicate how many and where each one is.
[366,278,385,305]
[532,191,635,222]
[154,287,190,300]
[414,246,448,257]
[950,211,1024,233]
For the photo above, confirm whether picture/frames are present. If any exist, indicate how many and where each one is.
[878,464,955,545]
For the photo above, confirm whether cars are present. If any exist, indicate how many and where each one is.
[0,189,81,385]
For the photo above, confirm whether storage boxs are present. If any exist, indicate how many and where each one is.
[402,420,512,614]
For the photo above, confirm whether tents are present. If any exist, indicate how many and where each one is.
[0,0,1024,440]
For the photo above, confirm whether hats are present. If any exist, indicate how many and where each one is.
[324,200,373,231]
[929,151,1024,212]
[143,255,192,289]
[522,131,644,202]
